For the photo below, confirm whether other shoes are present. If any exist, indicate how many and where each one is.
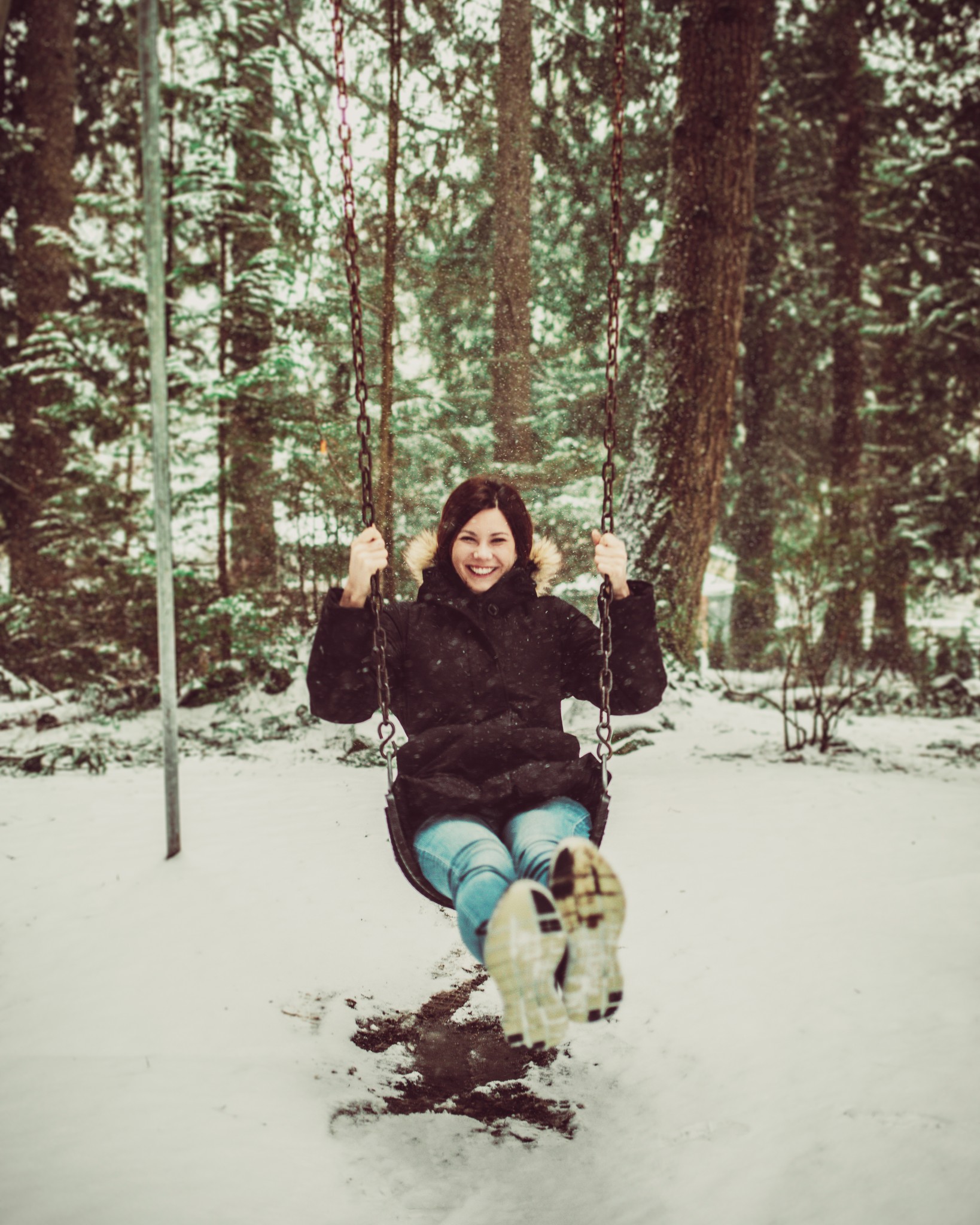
[481,876,567,1052]
[549,834,623,1020]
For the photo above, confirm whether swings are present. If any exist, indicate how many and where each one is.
[329,1,627,909]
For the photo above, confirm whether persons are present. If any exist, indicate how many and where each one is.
[306,476,667,1055]
